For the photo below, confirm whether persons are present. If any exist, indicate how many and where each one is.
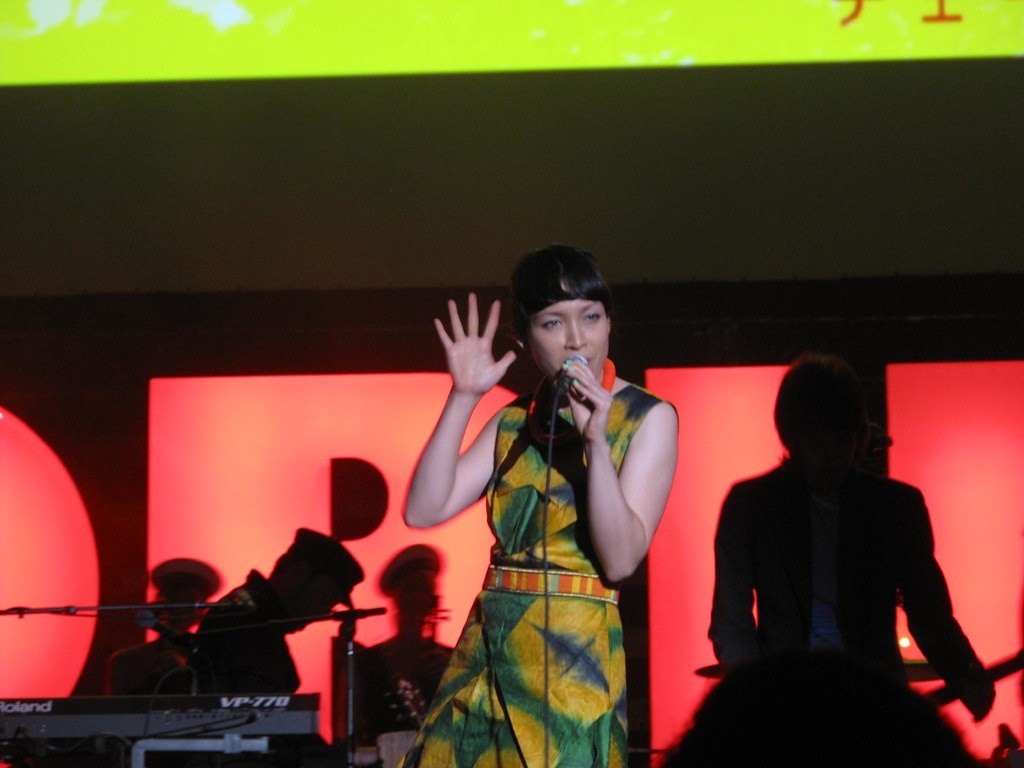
[396,245,681,768]
[661,359,997,768]
[104,558,220,697]
[364,544,453,696]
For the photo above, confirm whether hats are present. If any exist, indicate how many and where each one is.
[374,544,444,601]
[290,529,365,609]
[151,558,222,608]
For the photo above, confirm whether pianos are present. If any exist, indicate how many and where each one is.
[0,690,334,746]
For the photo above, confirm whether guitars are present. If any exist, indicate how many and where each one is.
[377,667,433,730]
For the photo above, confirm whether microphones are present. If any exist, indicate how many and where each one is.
[557,353,588,396]
